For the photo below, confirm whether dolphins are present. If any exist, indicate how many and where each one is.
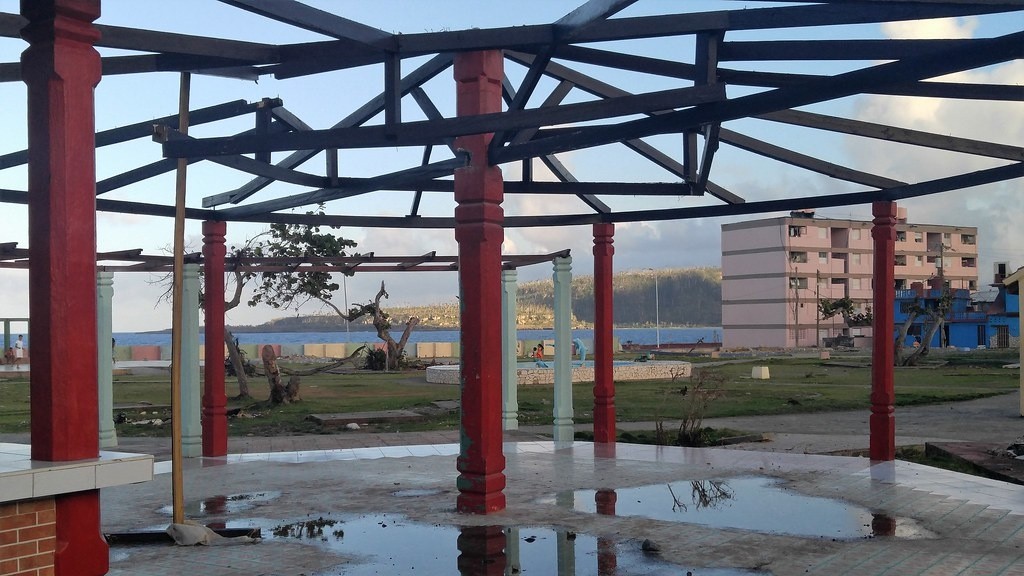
[536,360,549,368]
[572,338,588,365]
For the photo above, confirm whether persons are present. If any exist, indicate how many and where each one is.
[112,337,116,362]
[536,344,544,360]
[8,346,14,366]
[531,347,539,361]
[14,335,26,368]
